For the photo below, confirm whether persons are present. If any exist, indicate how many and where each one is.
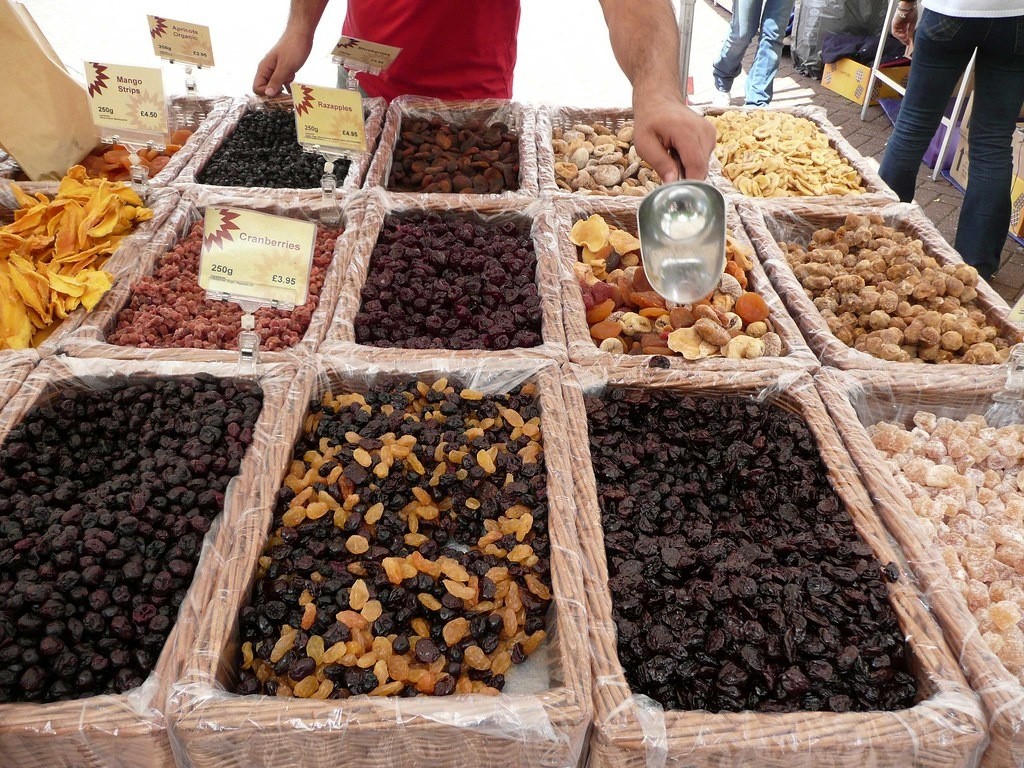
[879,0,1024,281]
[711,0,794,108]
[252,0,715,182]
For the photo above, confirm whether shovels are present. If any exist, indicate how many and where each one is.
[638,160,727,306]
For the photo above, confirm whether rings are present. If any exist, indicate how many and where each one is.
[899,28,902,31]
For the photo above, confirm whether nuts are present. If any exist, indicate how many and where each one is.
[0,109,1024,727]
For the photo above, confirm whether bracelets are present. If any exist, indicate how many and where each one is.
[895,5,916,18]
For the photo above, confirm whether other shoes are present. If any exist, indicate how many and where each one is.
[714,89,731,108]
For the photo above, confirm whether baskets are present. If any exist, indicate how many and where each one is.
[1,99,1024,768]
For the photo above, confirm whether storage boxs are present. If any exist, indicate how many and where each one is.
[821,56,911,105]
[950,123,1024,237]
